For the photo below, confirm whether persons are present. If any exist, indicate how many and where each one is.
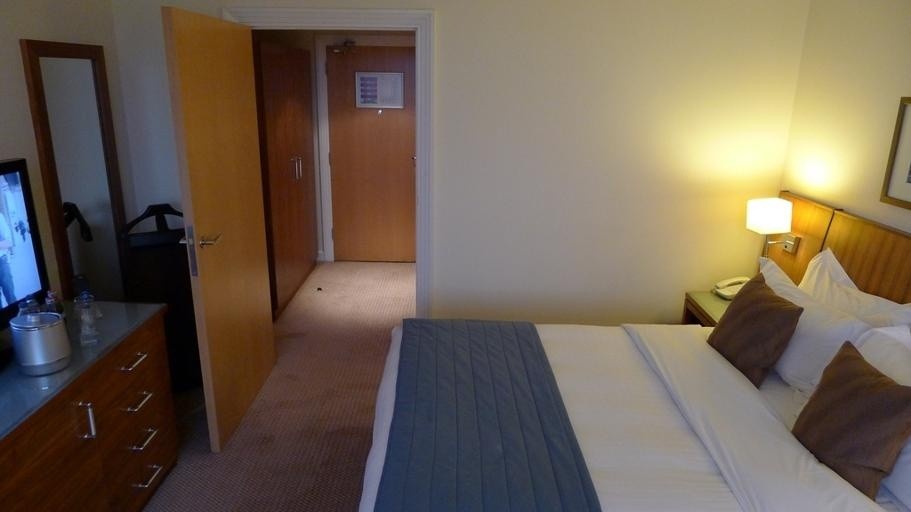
[0,212,17,308]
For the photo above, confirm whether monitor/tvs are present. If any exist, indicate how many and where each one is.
[0,157,51,333]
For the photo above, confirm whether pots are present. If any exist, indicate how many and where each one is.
[10,311,72,378]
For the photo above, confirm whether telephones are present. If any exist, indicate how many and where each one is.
[715,277,751,300]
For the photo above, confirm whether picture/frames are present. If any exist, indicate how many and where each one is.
[879,97,911,210]
[355,72,404,109]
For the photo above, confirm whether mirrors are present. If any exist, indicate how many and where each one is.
[19,38,133,301]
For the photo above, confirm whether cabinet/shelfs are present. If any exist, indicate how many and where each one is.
[253,41,317,322]
[0,299,179,512]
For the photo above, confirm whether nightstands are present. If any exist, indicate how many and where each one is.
[681,290,731,326]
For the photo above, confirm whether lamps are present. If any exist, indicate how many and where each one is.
[746,198,801,259]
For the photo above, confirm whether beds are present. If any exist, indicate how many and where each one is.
[357,318,911,512]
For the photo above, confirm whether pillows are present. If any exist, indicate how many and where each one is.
[707,273,804,388]
[854,327,911,512]
[760,256,866,397]
[798,247,911,328]
[791,340,911,502]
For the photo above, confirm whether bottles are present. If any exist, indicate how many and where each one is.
[18,289,102,350]
